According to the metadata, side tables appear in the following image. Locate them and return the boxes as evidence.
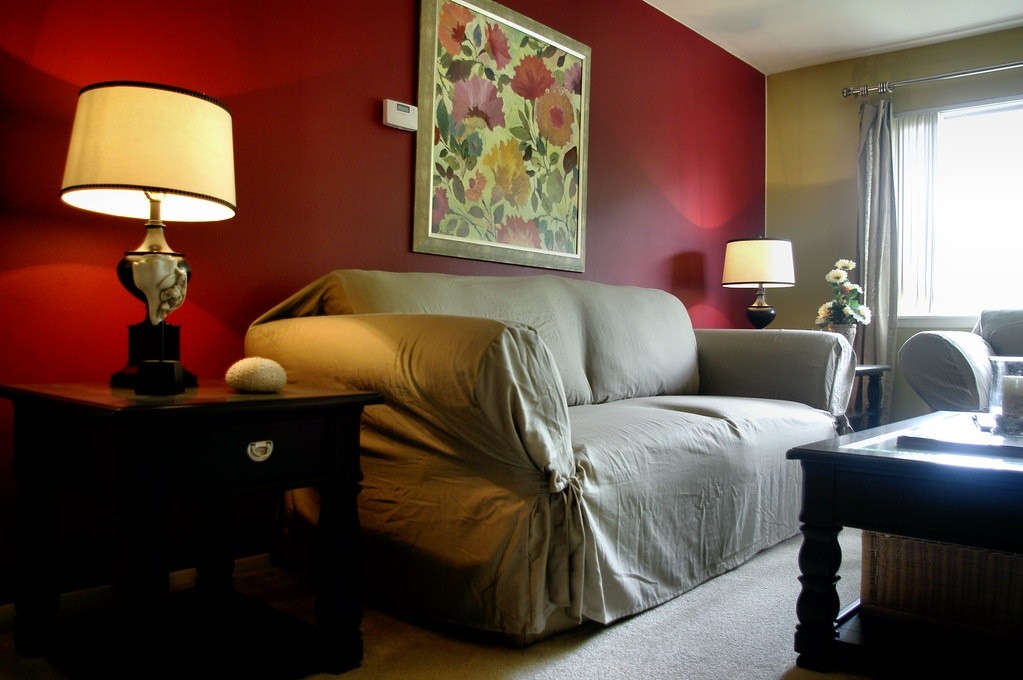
[845,365,892,427]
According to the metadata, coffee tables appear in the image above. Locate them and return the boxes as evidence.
[786,410,1023,679]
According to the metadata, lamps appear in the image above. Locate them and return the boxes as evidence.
[722,238,795,329]
[60,79,237,395]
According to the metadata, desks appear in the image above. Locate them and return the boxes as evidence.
[0,381,386,679]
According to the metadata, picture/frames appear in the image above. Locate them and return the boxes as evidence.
[412,1,590,275]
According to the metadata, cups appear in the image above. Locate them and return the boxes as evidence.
[988,356,1023,420]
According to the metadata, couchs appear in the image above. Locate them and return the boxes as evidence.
[244,269,857,648]
[897,309,1023,415]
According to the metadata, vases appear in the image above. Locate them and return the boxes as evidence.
[827,322,857,349]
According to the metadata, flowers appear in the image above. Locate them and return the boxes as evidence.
[813,258,870,329]
